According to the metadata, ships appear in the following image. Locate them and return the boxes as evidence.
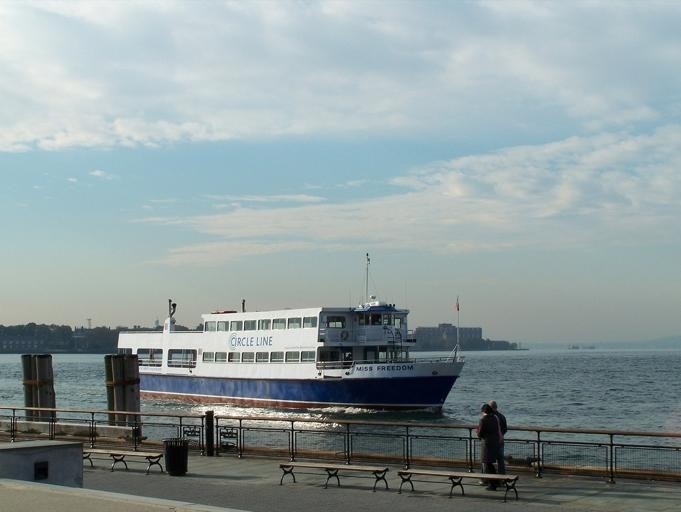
[117,253,468,412]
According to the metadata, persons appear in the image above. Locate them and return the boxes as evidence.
[478,401,507,491]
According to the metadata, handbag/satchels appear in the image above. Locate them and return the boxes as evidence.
[499,431,505,445]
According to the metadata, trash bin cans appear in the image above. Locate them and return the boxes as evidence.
[162,438,189,475]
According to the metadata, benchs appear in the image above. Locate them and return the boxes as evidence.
[279,461,520,502]
[82,449,164,475]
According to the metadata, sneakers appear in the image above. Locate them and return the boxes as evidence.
[479,481,488,486]
[485,486,496,491]
